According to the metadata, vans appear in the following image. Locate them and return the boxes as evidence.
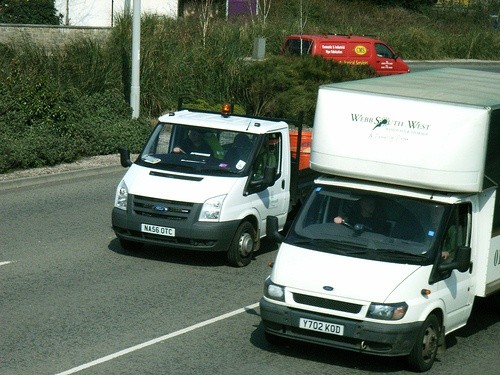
[280,35,410,75]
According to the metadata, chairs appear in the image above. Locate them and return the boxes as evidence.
[202,131,224,161]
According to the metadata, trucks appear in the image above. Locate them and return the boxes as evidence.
[259,65,500,375]
[111,93,323,267]
